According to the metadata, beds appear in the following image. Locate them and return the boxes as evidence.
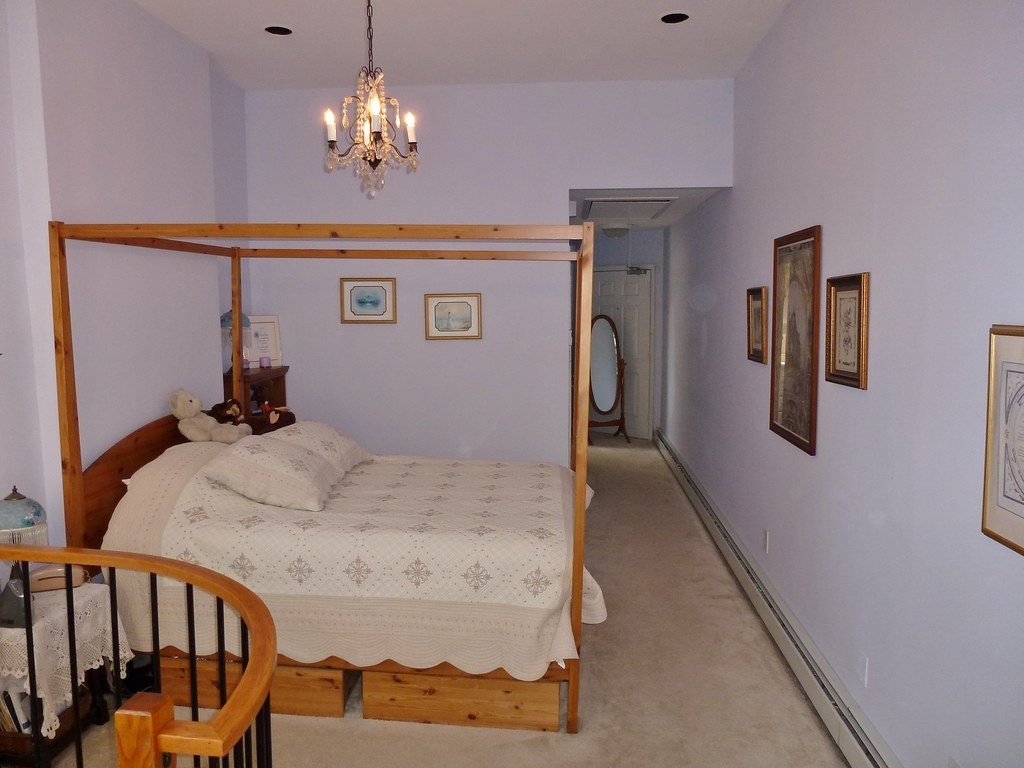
[84,409,609,732]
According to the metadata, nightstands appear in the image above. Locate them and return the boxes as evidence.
[0,582,136,768]
[224,366,290,420]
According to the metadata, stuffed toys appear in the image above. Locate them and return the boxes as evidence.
[201,398,296,436]
[168,389,252,445]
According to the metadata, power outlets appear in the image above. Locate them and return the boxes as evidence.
[763,531,768,553]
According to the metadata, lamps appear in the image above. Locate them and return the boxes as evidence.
[0,484,50,629]
[601,223,630,240]
[321,0,422,200]
[219,309,250,374]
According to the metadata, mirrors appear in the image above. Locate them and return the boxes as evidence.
[588,314,632,447]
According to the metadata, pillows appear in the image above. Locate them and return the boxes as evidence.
[204,418,373,513]
[122,440,229,508]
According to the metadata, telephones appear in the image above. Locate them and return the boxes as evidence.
[28,564,90,593]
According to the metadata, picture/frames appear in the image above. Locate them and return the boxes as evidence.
[424,293,482,340]
[340,277,397,324]
[825,271,870,390]
[745,286,768,365]
[981,324,1024,557]
[769,224,822,457]
